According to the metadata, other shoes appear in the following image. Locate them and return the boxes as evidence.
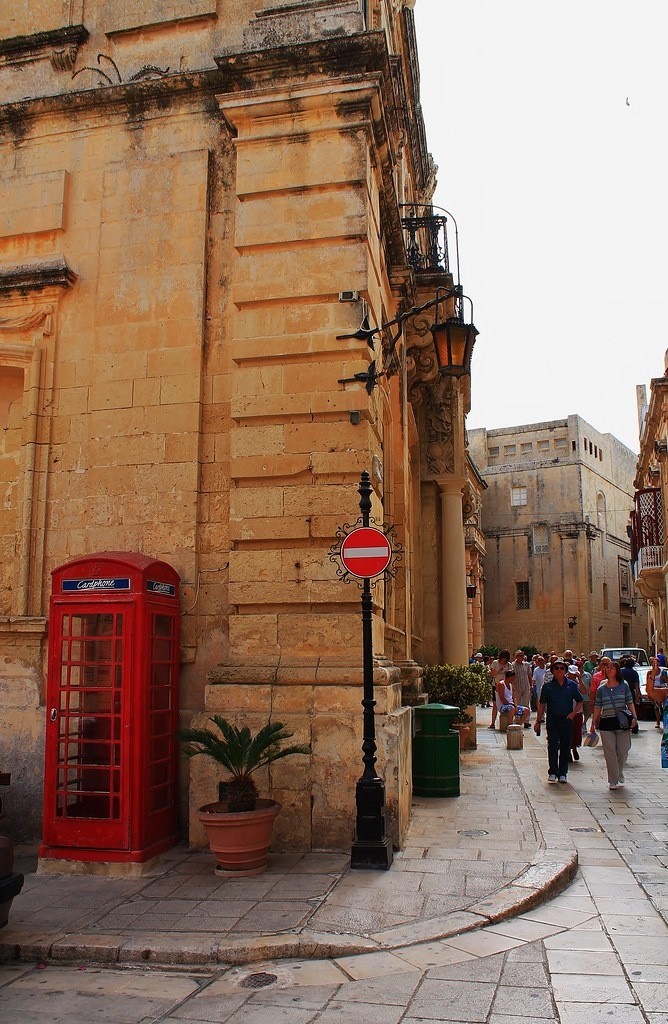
[568,749,579,763]
[548,774,556,783]
[559,775,567,783]
[618,774,624,783]
[524,723,531,727]
[609,782,617,790]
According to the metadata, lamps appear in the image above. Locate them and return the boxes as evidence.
[568,616,577,628]
[466,569,477,599]
[335,282,481,380]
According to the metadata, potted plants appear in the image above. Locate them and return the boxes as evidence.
[175,713,313,876]
[423,660,492,753]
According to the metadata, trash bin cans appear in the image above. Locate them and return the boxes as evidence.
[412,703,460,798]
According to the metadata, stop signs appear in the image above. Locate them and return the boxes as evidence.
[340,526,393,581]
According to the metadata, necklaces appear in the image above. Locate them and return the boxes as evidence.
[611,689,613,691]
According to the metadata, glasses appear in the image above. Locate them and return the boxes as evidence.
[518,655,525,657]
[554,666,565,670]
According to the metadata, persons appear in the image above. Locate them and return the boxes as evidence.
[590,661,637,790]
[534,660,583,782]
[468,647,668,768]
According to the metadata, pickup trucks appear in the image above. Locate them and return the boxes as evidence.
[596,647,668,700]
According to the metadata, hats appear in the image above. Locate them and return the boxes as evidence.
[550,660,568,675]
[568,664,578,674]
[588,651,599,658]
[514,650,524,657]
[474,653,482,658]
[583,732,599,747]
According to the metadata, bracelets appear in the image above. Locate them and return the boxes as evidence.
[537,720,541,723]
[633,717,637,720]
[650,677,655,682]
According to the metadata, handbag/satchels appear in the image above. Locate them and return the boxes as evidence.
[616,710,630,729]
